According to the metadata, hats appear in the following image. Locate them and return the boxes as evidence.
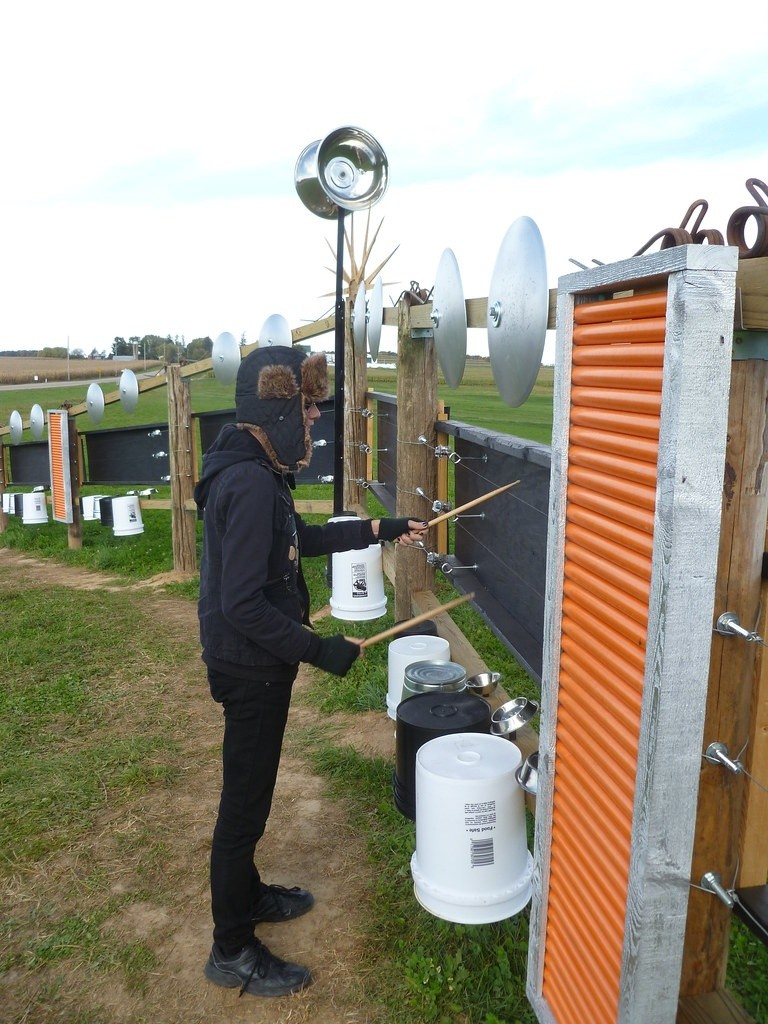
[235,345,328,473]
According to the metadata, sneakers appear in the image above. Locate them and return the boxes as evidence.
[252,881,313,922]
[202,937,311,998]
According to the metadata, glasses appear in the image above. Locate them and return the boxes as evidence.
[304,398,312,412]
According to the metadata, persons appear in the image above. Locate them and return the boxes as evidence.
[194,346,430,997]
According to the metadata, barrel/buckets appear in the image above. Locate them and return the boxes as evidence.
[332,511,358,517]
[1,492,23,517]
[79,494,119,526]
[327,516,362,523]
[392,695,492,822]
[400,660,468,702]
[386,634,450,722]
[393,618,438,640]
[326,553,332,588]
[22,493,49,525]
[328,544,388,621]
[111,495,145,537]
[409,730,535,925]
